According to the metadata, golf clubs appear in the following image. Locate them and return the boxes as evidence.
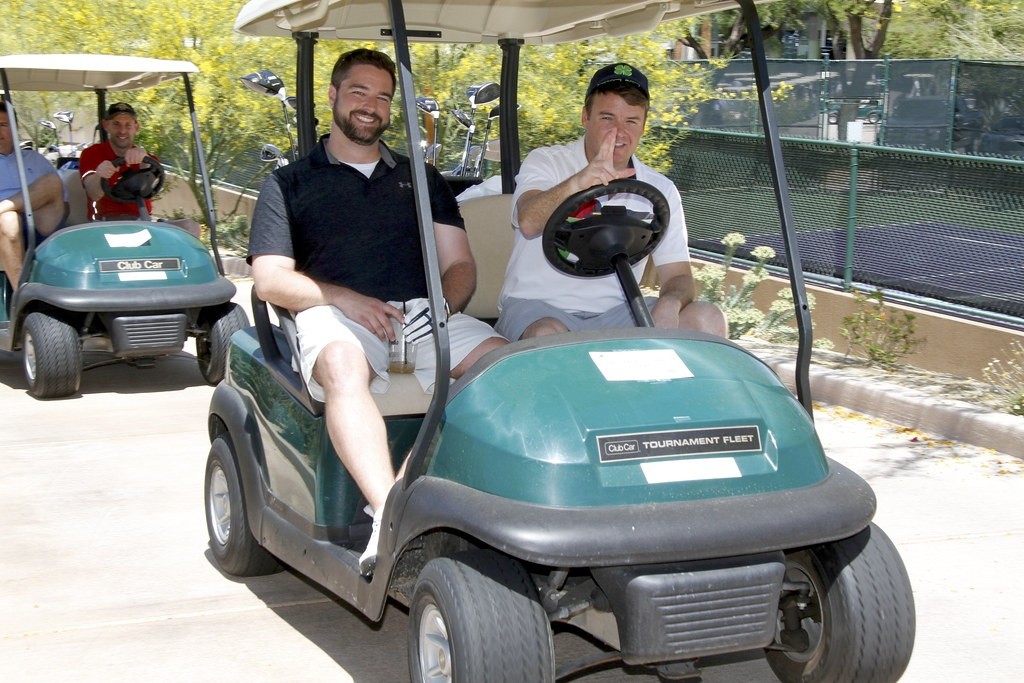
[414,81,522,177]
[39,110,74,158]
[241,70,320,167]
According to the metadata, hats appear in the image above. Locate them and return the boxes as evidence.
[586,63,648,97]
[108,102,135,119]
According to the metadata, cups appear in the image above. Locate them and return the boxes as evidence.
[386,315,416,374]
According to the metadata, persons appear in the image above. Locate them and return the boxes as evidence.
[79,102,201,239]
[0,101,71,292]
[246,49,512,575]
[493,62,729,343]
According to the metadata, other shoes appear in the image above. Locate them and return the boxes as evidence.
[359,502,384,575]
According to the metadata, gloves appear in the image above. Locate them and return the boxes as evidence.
[402,296,451,345]
